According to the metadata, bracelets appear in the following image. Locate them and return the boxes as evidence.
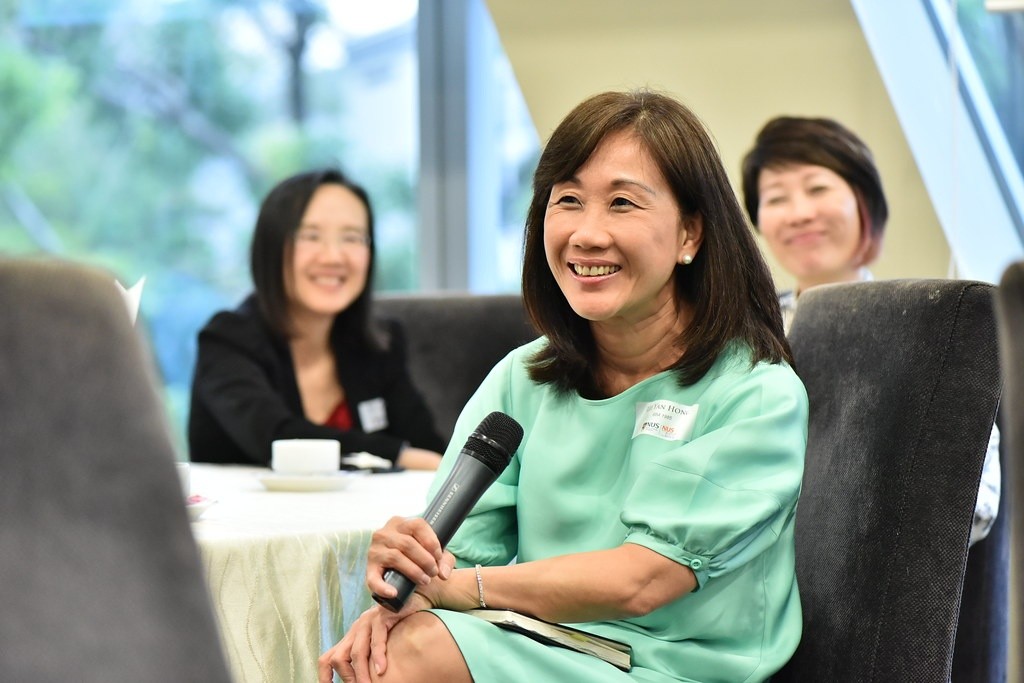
[475,564,488,609]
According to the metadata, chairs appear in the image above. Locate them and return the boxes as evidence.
[0,256,231,683]
[369,293,545,447]
[784,262,1023,683]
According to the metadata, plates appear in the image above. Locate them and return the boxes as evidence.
[179,499,218,522]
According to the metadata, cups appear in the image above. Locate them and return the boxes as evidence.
[271,439,340,477]
[260,476,343,492]
[175,461,191,500]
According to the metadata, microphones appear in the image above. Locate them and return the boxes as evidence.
[370,411,524,613]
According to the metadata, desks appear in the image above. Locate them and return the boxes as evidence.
[175,463,439,683]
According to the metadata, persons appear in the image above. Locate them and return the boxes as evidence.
[187,169,450,475]
[740,116,1003,551]
[317,90,810,683]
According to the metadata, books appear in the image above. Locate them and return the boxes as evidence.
[463,606,633,673]
